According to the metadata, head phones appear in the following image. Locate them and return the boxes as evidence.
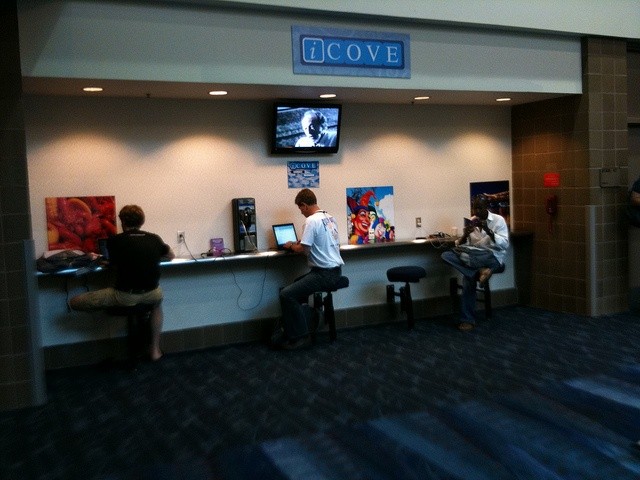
[433,232,445,238]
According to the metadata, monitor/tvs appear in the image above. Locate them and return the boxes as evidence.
[265,98,343,155]
[272,224,298,246]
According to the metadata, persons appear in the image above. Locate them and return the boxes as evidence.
[632,177,640,206]
[348,203,372,245]
[295,110,338,148]
[278,187,345,354]
[442,193,510,333]
[69,204,176,362]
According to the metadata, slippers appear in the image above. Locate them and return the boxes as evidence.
[150,345,166,362]
[479,268,495,288]
[459,322,476,330]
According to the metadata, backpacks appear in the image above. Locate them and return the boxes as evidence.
[36,249,101,275]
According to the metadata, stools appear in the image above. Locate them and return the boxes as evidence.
[476,262,505,321]
[387,266,425,330]
[321,276,349,343]
[121,304,153,364]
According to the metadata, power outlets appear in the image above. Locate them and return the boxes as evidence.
[177,230,185,243]
[416,217,422,228]
